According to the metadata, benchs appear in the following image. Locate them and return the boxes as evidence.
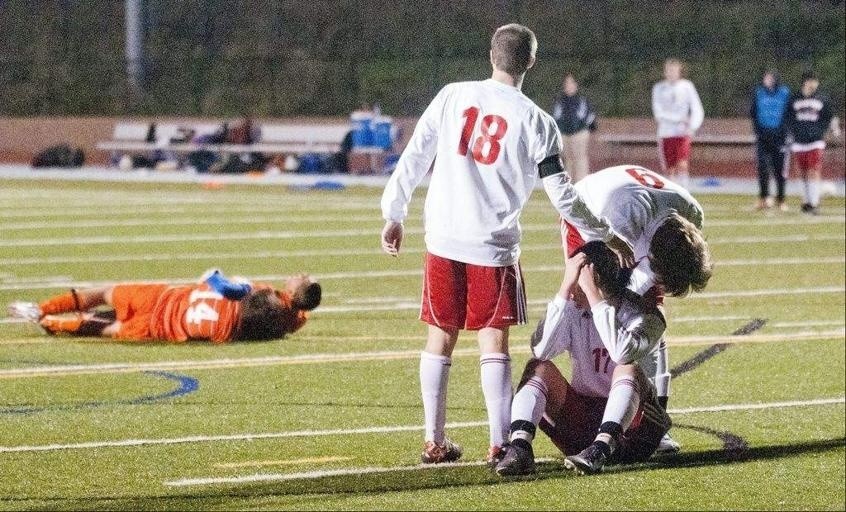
[97,124,352,173]
[593,133,846,185]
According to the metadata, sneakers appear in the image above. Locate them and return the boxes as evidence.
[564,444,606,475]
[486,441,511,467]
[7,301,45,325]
[421,437,464,463]
[495,444,536,475]
[656,432,680,452]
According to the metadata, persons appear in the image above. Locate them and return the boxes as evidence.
[560,161,712,453]
[378,22,637,465]
[490,240,672,478]
[552,68,597,178]
[778,71,841,212]
[652,58,707,177]
[2,281,290,344]
[196,264,323,336]
[364,102,392,151]
[348,99,372,148]
[748,70,794,215]
